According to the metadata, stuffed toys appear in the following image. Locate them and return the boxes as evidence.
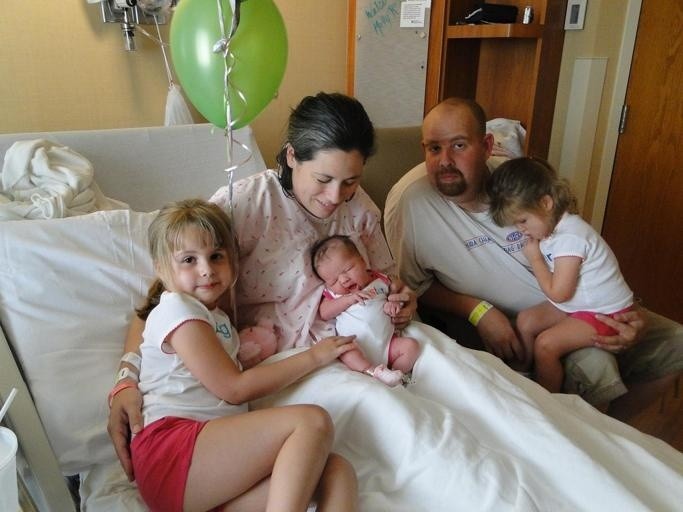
[238,316,279,372]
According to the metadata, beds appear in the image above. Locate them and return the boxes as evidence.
[1,122,683,512]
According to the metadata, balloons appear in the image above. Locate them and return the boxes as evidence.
[168,0,288,135]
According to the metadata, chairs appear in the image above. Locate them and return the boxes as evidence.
[360,126,426,259]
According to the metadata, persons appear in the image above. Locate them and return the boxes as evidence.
[130,196,358,512]
[104,89,417,481]
[310,231,421,387]
[398,96,682,423]
[488,154,635,393]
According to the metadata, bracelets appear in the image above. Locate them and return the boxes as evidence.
[107,381,139,408]
[118,352,142,369]
[113,368,139,383]
[468,301,494,326]
